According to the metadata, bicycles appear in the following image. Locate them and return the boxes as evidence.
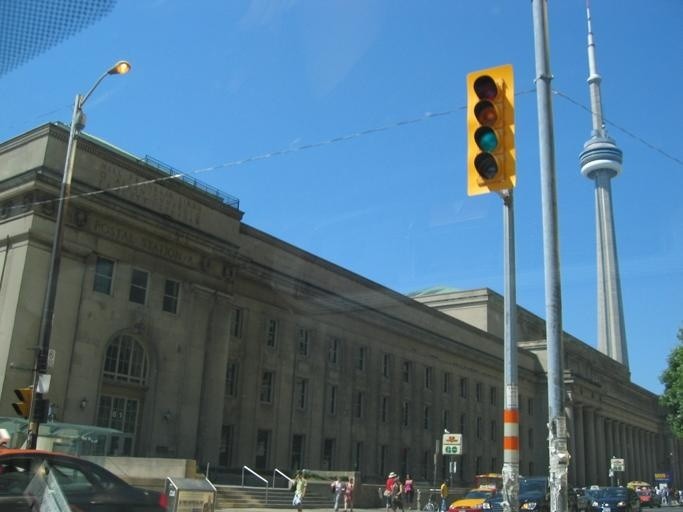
[422,492,438,512]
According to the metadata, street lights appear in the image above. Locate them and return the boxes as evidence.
[24,59,131,452]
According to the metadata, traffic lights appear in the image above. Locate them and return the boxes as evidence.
[11,387,32,419]
[473,74,501,181]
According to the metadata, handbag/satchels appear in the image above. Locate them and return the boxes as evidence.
[384,490,391,496]
[332,486,335,492]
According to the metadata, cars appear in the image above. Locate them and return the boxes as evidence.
[0,448,167,511]
[446,473,661,512]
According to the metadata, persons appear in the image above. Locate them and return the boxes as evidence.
[330,474,346,511]
[655,485,681,506]
[384,472,397,509]
[342,476,355,512]
[439,476,450,512]
[390,476,404,511]
[0,427,11,474]
[291,471,308,512]
[403,472,416,511]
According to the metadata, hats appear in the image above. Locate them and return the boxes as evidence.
[295,470,301,475]
[389,472,397,478]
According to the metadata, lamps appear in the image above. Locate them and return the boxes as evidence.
[79,396,88,409]
